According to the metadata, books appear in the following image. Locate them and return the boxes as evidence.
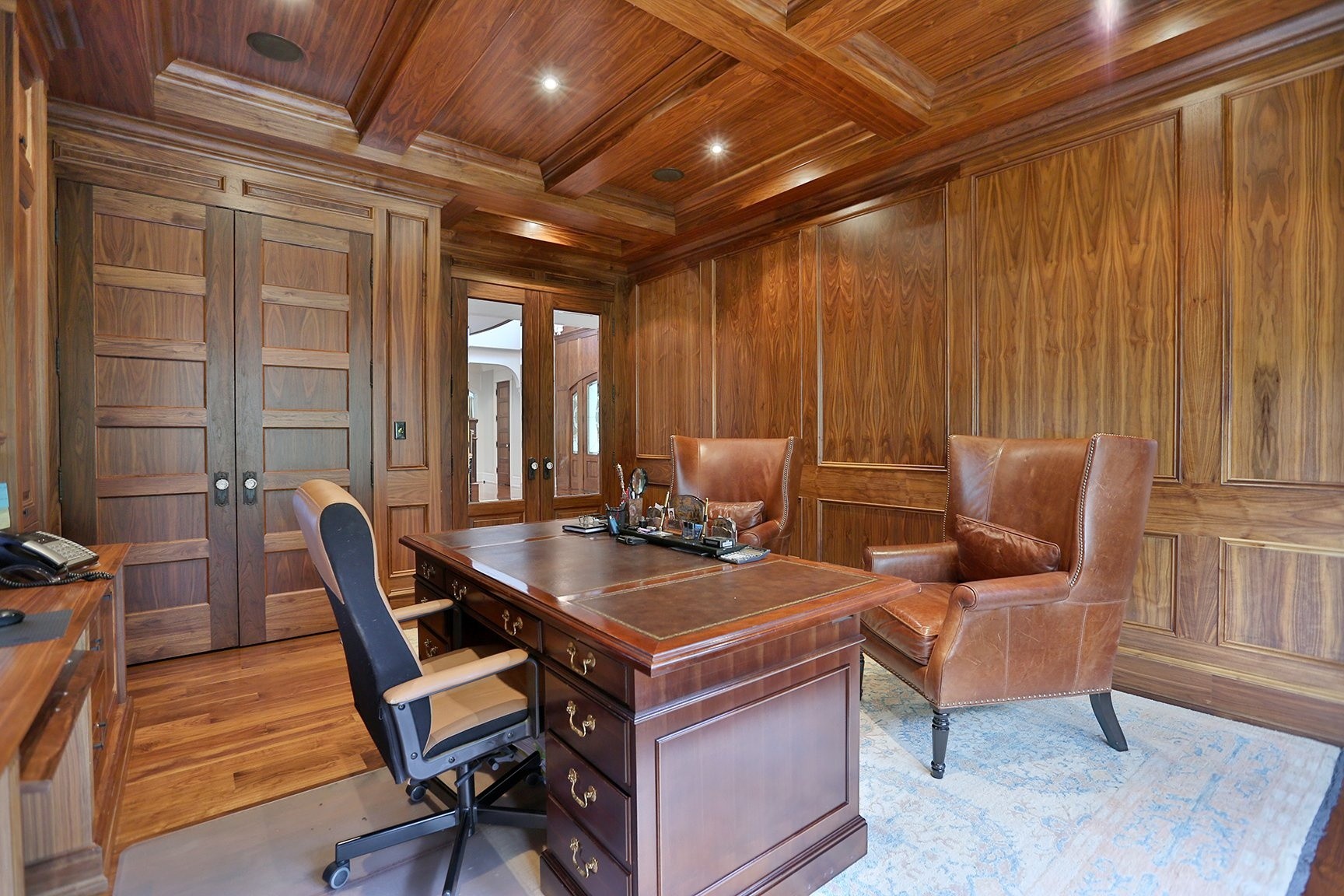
[562,522,606,534]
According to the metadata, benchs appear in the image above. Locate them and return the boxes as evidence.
[392,515,924,896]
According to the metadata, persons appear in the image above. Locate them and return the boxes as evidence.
[669,511,674,520]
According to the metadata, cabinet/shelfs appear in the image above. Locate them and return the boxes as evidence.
[0,539,142,896]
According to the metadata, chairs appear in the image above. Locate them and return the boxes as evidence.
[667,435,809,554]
[290,476,545,896]
[844,431,1164,783]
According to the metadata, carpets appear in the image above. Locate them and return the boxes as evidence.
[122,656,1344,896]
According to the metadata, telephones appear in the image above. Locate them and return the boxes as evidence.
[0,530,100,582]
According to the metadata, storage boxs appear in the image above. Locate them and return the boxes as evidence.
[620,497,643,526]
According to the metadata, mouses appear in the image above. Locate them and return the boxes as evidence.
[0,609,25,627]
